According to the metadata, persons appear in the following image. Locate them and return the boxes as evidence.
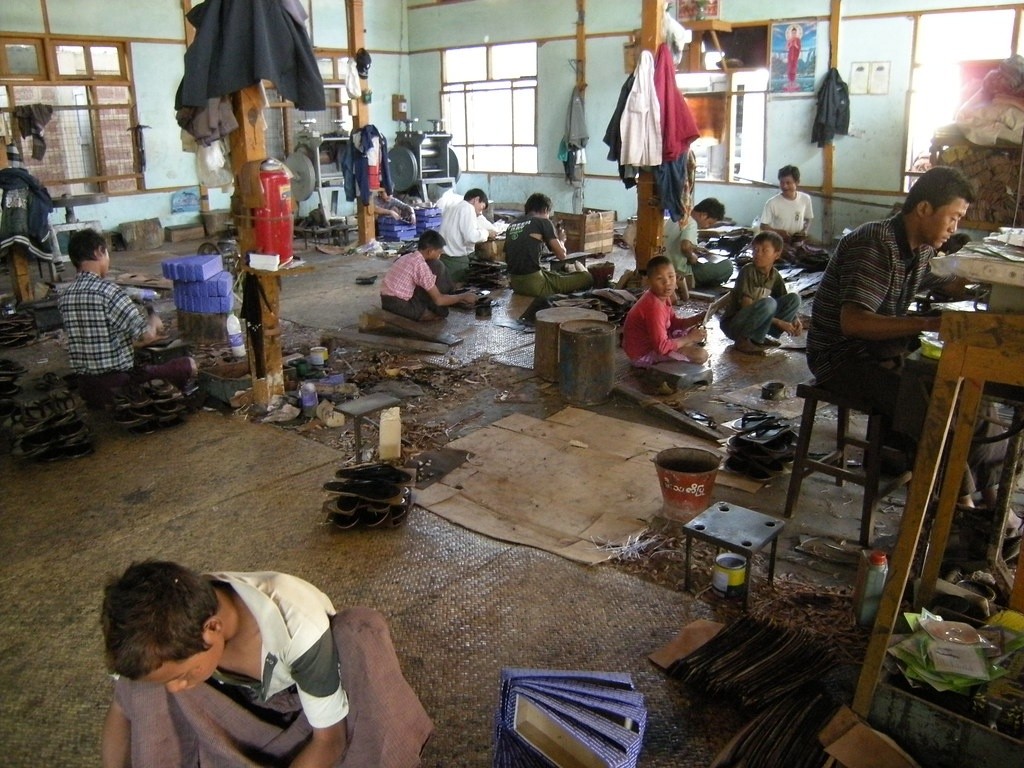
[720,232,803,355]
[623,259,709,368]
[373,180,416,234]
[503,194,592,297]
[663,198,733,289]
[760,165,813,261]
[102,561,432,768]
[380,230,479,321]
[59,227,198,403]
[807,168,1024,561]
[439,189,497,282]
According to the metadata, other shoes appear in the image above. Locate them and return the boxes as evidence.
[1,313,184,466]
[759,337,777,347]
[725,411,798,483]
[736,339,766,354]
[419,308,443,321]
[655,380,672,396]
[1001,517,1023,562]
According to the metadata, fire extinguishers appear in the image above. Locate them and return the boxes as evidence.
[367,136,381,188]
[253,159,293,263]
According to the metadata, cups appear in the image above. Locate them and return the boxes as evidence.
[301,383,318,416]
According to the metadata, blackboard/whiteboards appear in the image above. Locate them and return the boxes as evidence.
[698,20,770,73]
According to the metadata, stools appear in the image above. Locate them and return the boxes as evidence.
[682,500,785,613]
[305,225,356,251]
[783,378,917,549]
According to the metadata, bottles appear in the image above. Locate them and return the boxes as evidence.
[853,549,888,624]
[226,312,247,358]
[126,287,160,301]
[712,552,747,600]
[751,219,760,237]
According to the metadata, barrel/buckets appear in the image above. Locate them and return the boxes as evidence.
[650,448,723,522]
[288,360,309,379]
[557,319,617,408]
[588,264,614,289]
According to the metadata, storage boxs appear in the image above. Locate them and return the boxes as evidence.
[556,208,613,254]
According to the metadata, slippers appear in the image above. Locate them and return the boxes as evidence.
[324,463,406,530]
[686,408,718,428]
